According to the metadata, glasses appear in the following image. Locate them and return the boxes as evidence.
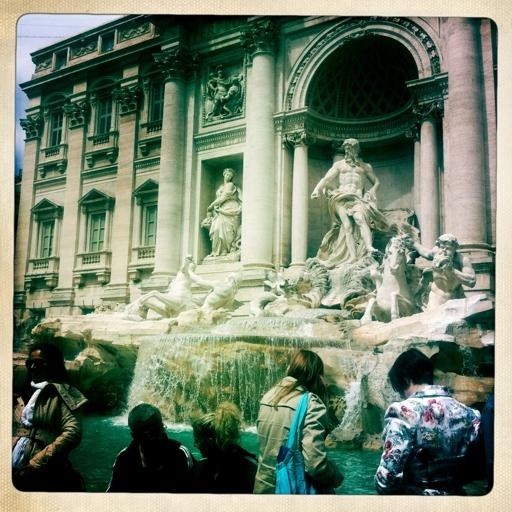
[25,358,47,369]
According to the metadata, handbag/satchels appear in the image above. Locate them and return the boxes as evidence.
[274,447,338,494]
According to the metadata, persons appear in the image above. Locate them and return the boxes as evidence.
[13,343,83,491]
[205,167,241,257]
[400,230,477,311]
[478,360,493,488]
[206,67,241,122]
[187,262,243,327]
[373,348,479,495]
[108,404,188,486]
[183,401,258,492]
[311,136,380,264]
[253,347,343,494]
